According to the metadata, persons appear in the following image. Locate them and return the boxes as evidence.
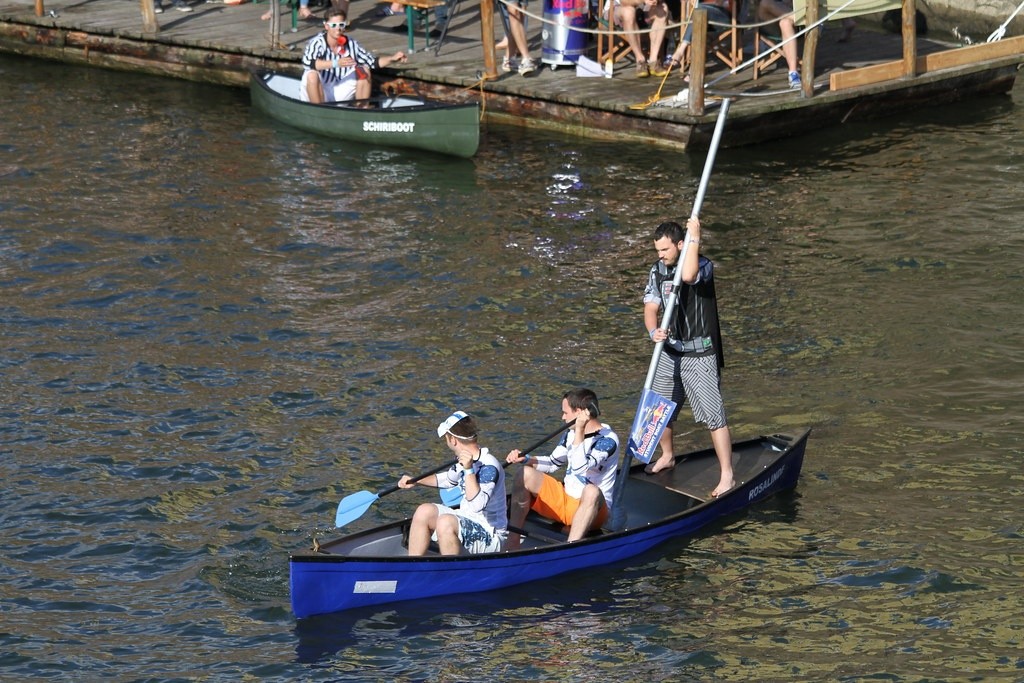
[154,0,193,13]
[494,0,856,89]
[397,411,507,556]
[261,0,449,37]
[505,388,620,550]
[300,7,409,108]
[644,216,736,497]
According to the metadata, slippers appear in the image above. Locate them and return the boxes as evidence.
[375,6,405,17]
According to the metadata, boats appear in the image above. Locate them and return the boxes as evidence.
[248,67,484,161]
[291,421,811,620]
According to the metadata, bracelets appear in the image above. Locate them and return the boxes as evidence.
[520,454,531,464]
[689,239,701,245]
[649,328,658,339]
[464,468,475,476]
[332,59,337,68]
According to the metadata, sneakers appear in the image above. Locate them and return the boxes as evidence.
[788,71,801,89]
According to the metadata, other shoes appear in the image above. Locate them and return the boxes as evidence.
[636,60,649,78]
[392,24,408,32]
[521,58,539,78]
[670,59,681,71]
[502,56,513,72]
[176,1,192,12]
[650,61,670,77]
[154,3,163,13]
[429,28,448,36]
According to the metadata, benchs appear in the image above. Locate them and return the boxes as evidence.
[385,0,446,53]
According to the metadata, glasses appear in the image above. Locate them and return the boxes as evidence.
[323,22,345,29]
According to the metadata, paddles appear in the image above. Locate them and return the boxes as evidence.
[334,459,460,526]
[501,418,576,468]
[607,95,730,535]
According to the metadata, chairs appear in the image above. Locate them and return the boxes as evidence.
[596,0,804,79]
